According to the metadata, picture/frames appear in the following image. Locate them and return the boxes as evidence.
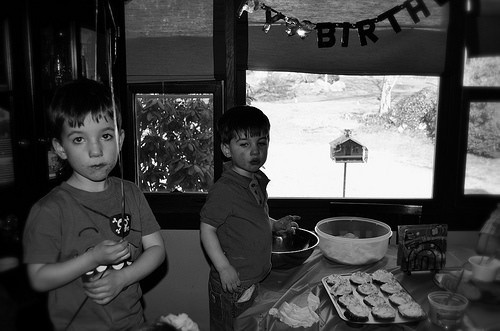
[124,79,224,208]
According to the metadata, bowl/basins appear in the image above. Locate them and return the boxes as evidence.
[314,217,393,266]
[469,256,500,282]
[271,227,319,270]
[428,291,468,328]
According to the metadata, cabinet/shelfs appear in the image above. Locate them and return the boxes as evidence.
[0,0,132,254]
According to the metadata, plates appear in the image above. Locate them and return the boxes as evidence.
[443,278,481,299]
[433,273,460,290]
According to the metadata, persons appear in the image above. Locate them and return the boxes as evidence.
[476,205,500,257]
[200,106,301,331]
[20,79,165,331]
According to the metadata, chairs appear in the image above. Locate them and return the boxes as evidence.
[330,201,422,227]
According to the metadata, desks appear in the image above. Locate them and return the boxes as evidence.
[236,228,500,331]
